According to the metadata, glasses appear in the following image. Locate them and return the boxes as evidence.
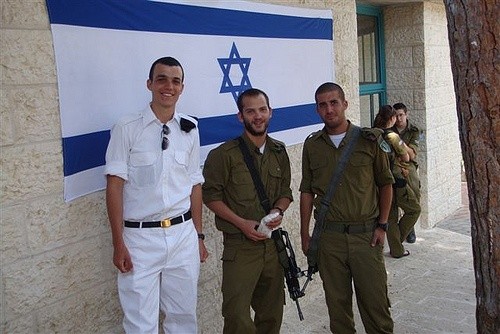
[389,105,395,113]
[160,125,173,150]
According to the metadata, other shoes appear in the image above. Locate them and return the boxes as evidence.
[403,248,410,256]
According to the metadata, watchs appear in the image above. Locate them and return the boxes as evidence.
[376,222,389,231]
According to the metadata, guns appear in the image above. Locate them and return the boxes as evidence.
[254,221,309,322]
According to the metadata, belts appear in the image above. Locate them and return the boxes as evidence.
[124,211,193,228]
[323,222,379,234]
[224,233,246,240]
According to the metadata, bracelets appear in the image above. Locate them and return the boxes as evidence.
[273,207,284,217]
[198,233,205,240]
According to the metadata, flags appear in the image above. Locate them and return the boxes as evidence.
[46,0,334,203]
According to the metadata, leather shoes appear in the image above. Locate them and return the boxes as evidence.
[407,232,416,243]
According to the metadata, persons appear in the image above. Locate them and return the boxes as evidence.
[392,102,423,243]
[104,56,208,333]
[298,81,396,334]
[202,88,294,334]
[372,104,422,259]
